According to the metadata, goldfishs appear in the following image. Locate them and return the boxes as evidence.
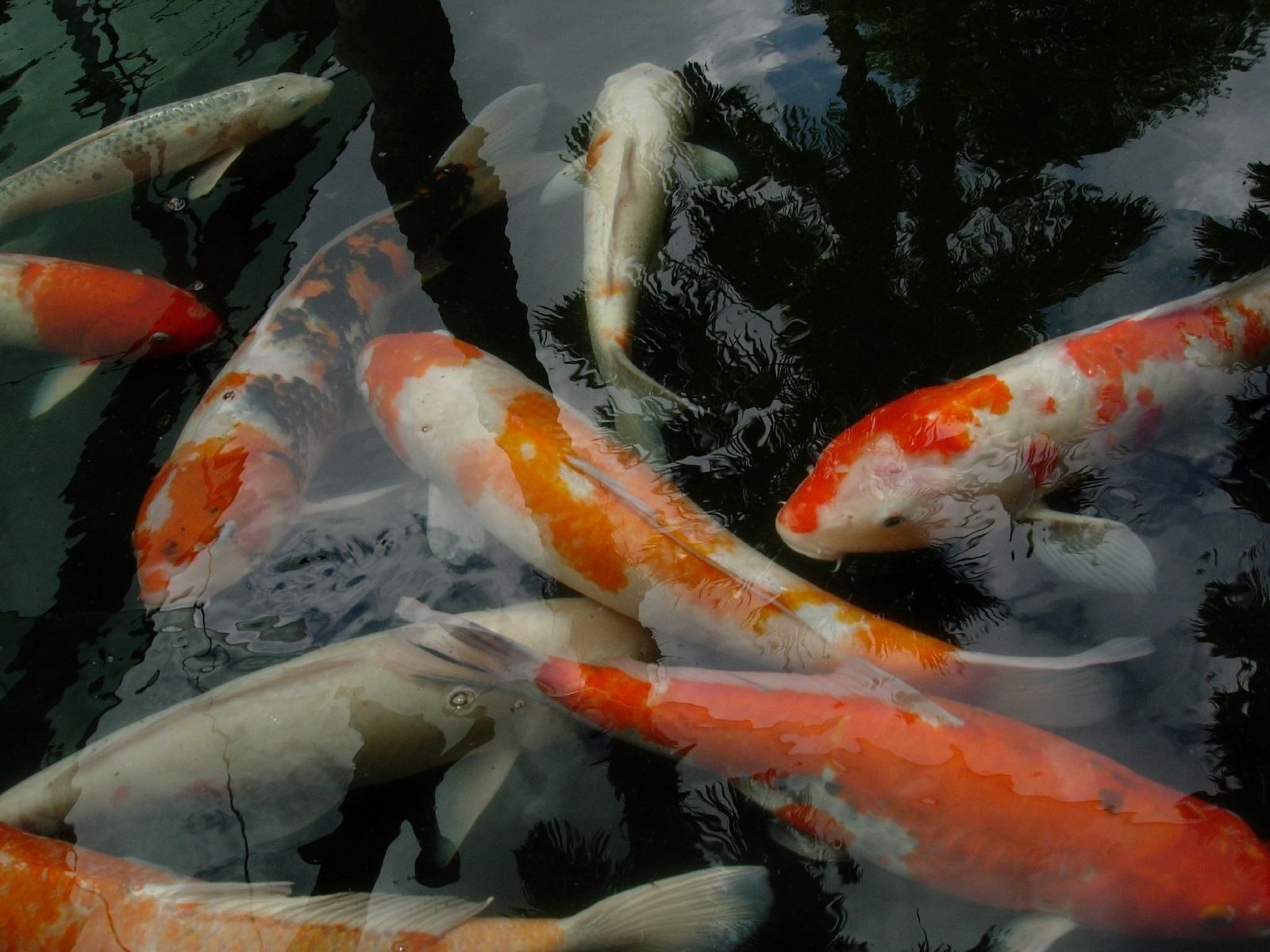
[0,63,1270,952]
